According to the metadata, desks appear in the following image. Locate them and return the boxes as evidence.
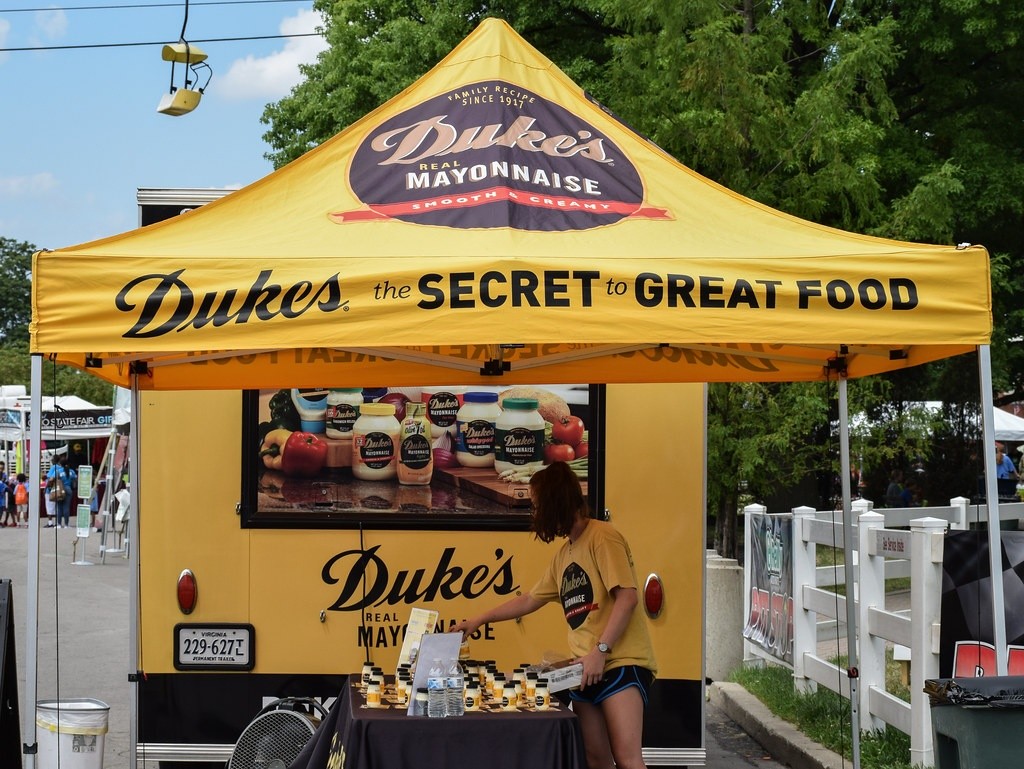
[280,673,587,769]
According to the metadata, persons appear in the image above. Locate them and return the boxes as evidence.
[447,461,658,768]
[995,446,1020,480]
[0,461,6,481]
[46,459,77,528]
[850,463,859,500]
[900,478,918,507]
[13,473,28,527]
[0,472,13,523]
[86,480,98,527]
[1019,448,1024,482]
[1,480,16,527]
[40,475,47,487]
[43,454,62,527]
[886,469,904,496]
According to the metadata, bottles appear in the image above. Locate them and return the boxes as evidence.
[457,631,471,660]
[287,386,546,487]
[358,656,551,717]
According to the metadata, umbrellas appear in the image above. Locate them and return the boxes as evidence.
[828,401,1024,442]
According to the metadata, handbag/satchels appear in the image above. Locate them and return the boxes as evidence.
[48,479,66,503]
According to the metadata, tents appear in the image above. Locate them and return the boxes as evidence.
[23,19,1009,769]
[0,395,113,527]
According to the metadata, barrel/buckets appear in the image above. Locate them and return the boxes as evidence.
[35,695,110,769]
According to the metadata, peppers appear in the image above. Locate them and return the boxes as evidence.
[259,390,328,482]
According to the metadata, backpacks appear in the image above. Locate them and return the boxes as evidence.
[15,484,27,504]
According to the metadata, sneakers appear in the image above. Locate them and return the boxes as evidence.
[1,521,16,527]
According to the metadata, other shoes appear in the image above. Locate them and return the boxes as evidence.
[54,524,71,529]
[17,522,29,528]
[44,524,53,527]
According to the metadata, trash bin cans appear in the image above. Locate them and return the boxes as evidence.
[36,696,110,769]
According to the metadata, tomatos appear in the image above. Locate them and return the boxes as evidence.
[544,417,588,463]
